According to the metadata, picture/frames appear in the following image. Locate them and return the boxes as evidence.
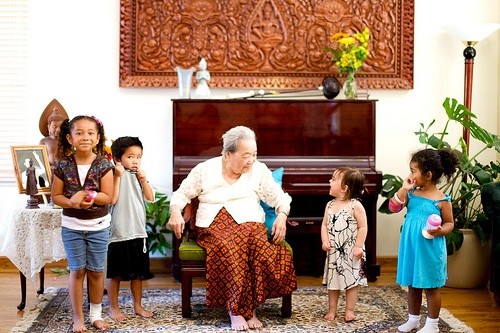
[10,145,53,194]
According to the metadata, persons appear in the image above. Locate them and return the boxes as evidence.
[106,136,155,320]
[320,166,368,322]
[26,159,38,199]
[389,148,461,333]
[39,99,69,183]
[196,58,210,97]
[169,125,297,330]
[51,116,116,332]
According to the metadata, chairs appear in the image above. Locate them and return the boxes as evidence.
[173,170,293,318]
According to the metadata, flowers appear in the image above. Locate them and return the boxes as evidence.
[92,116,103,127]
[326,26,371,96]
[93,145,112,161]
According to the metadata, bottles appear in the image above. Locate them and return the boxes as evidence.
[422,214,442,240]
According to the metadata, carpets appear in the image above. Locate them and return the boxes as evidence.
[9,286,474,333]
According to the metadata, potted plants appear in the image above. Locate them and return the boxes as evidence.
[379,96,500,288]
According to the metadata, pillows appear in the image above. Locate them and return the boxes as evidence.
[260,167,284,239]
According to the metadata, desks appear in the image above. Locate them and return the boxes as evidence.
[0,204,107,309]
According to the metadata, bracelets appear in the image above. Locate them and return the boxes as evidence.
[279,211,288,216]
[394,192,406,204]
[85,190,97,203]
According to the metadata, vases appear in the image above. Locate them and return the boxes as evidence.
[343,70,358,98]
